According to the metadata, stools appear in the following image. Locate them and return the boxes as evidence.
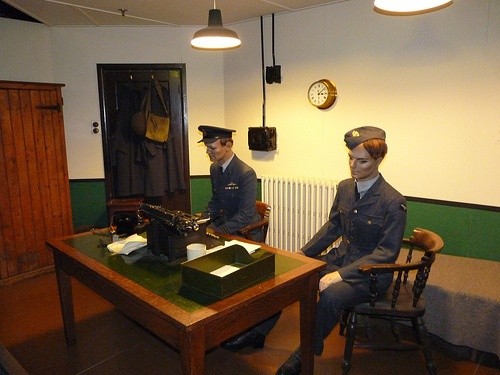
[107,198,144,226]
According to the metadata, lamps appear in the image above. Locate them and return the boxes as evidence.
[373,0,455,16]
[191,0,242,52]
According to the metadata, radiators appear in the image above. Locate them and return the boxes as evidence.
[261,175,342,256]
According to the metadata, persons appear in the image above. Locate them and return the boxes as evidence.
[198,125,264,242]
[220,126,408,375]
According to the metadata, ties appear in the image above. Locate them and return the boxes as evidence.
[355,182,360,202]
[219,167,223,178]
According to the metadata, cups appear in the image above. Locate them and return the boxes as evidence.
[186,244,206,260]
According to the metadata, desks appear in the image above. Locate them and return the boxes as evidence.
[45,217,327,375]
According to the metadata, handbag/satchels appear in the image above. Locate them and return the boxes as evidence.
[130,71,170,142]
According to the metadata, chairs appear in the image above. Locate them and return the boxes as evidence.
[195,200,271,244]
[340,228,444,375]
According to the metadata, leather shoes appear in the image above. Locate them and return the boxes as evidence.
[220,329,264,351]
[275,348,302,375]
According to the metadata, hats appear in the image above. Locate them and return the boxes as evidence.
[197,125,236,143]
[344,126,386,150]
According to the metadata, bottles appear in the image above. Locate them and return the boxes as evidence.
[116,216,134,236]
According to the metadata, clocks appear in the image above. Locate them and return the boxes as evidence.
[308,79,337,110]
